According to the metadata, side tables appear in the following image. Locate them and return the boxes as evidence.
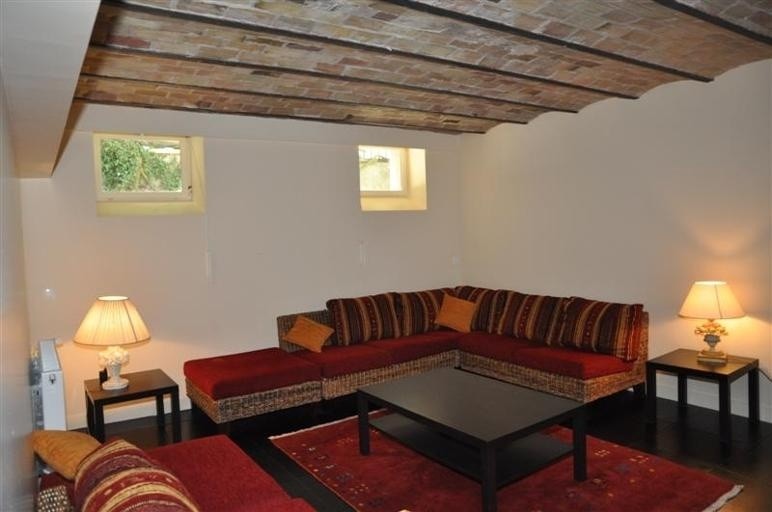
[646,348,760,442]
[84,368,182,445]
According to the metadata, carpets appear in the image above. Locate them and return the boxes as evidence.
[269,409,745,512]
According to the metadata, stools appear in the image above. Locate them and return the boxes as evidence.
[183,347,322,424]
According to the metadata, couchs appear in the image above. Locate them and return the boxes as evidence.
[276,286,649,421]
[36,435,316,512]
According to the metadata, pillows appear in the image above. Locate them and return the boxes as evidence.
[325,291,400,343]
[432,291,476,334]
[463,284,643,362]
[388,284,456,336]
[282,313,335,353]
[28,430,201,512]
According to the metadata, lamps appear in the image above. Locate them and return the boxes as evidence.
[73,295,150,390]
[677,281,745,355]
